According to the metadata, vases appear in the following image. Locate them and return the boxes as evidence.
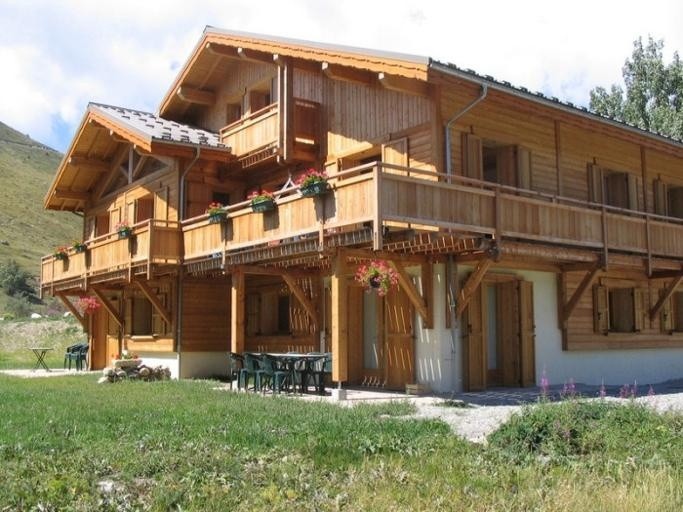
[301,185,328,196]
[370,274,381,287]
[209,215,227,224]
[76,246,84,251]
[251,201,274,214]
[57,254,64,260]
[118,231,131,239]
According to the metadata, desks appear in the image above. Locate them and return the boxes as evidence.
[28,347,54,372]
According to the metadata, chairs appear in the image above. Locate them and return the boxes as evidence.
[231,349,332,395]
[64,343,82,371]
[69,343,89,371]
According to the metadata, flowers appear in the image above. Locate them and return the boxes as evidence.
[72,239,87,248]
[205,202,227,215]
[297,168,330,185]
[54,247,66,256]
[247,190,275,205]
[114,220,132,232]
[354,261,401,299]
